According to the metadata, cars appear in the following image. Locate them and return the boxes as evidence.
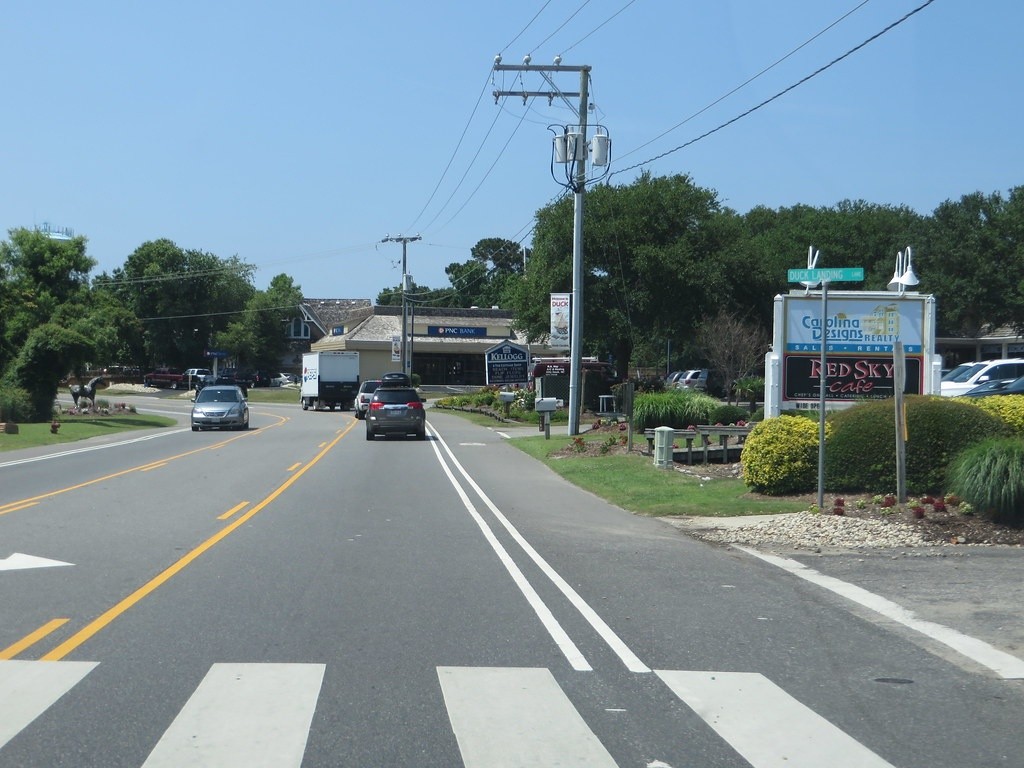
[190,384,251,432]
[938,356,1024,400]
[215,367,302,389]
[666,368,756,401]
[354,379,382,421]
[182,369,212,382]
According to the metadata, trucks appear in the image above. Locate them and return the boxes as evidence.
[297,351,358,412]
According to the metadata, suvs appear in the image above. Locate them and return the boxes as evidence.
[362,383,426,441]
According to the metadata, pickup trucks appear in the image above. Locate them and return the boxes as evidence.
[143,367,198,390]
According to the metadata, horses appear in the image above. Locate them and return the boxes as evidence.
[69,376,106,406]
